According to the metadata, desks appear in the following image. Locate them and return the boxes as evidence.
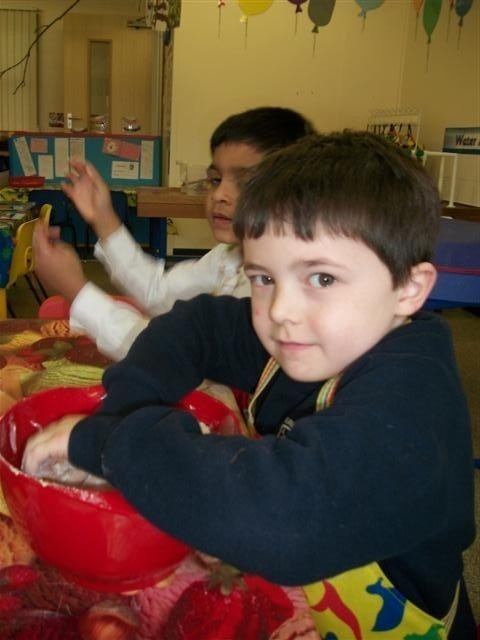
[137,184,214,262]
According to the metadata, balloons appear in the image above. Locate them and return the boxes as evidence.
[236,0,479,47]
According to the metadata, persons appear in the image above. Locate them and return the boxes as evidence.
[19,129,479,639]
[30,106,320,366]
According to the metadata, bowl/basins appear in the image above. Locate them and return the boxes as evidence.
[1,383,244,595]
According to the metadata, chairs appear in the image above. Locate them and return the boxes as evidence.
[6,203,54,320]
